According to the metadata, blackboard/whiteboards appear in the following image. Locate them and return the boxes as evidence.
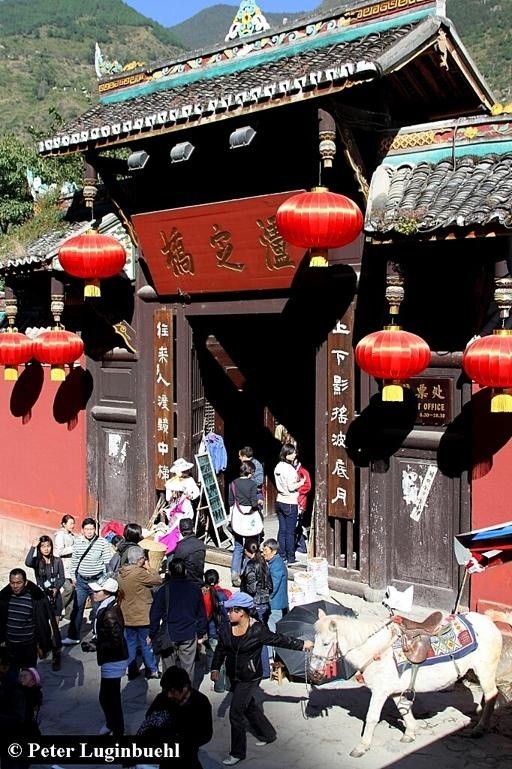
[194,451,228,529]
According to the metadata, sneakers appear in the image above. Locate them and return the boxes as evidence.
[221,748,247,765]
[59,634,82,645]
[255,736,277,747]
[231,570,244,588]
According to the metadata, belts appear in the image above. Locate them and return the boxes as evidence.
[76,571,103,582]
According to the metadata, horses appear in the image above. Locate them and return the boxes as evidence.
[308,608,503,759]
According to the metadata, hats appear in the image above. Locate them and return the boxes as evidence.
[168,458,195,473]
[86,575,120,594]
[218,589,256,610]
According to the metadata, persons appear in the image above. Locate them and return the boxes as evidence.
[160,457,201,527]
[1,511,314,769]
[237,445,266,496]
[227,459,266,588]
[273,443,299,565]
[292,449,312,554]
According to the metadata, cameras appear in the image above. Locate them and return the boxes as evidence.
[44,585,55,603]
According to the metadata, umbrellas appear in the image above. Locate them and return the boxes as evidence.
[273,600,360,686]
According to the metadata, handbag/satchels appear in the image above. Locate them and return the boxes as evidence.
[230,499,264,537]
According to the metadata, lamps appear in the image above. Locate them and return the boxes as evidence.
[125,140,159,176]
[227,123,254,149]
[168,135,196,164]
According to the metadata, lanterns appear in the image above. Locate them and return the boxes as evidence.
[351,322,436,401]
[29,323,89,381]
[56,229,128,298]
[462,326,512,414]
[271,184,365,268]
[0,325,35,382]
